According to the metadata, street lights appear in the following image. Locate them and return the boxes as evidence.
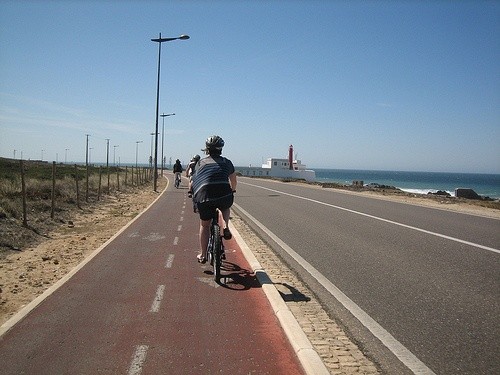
[150,32,190,192]
[150,132,160,169]
[159,112,175,178]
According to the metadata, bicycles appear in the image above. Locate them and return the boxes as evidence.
[197,190,237,283]
[175,173,181,189]
[187,176,197,213]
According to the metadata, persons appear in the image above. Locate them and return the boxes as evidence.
[185,154,200,198]
[190,136,236,264]
[173,158,182,187]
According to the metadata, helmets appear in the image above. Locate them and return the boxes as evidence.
[205,135,225,153]
[193,154,200,160]
[176,159,179,163]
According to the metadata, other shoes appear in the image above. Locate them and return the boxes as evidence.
[197,254,207,263]
[223,227,232,240]
